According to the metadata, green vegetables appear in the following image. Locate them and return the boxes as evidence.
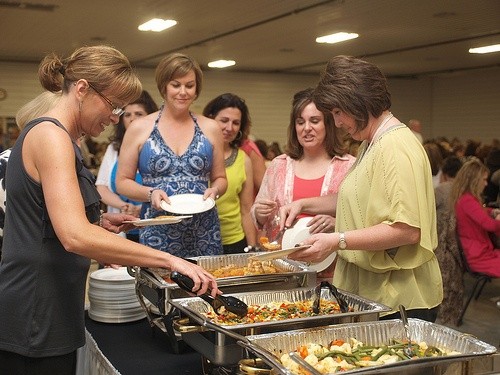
[205,303,313,322]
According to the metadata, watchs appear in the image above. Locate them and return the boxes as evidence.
[338,233,347,250]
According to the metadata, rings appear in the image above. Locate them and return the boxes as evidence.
[322,223,326,228]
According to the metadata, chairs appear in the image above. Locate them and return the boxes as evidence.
[456,229,497,326]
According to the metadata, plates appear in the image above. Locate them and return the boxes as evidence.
[160,193,215,214]
[281,217,336,273]
[87,266,164,324]
[122,214,193,226]
[247,244,311,262]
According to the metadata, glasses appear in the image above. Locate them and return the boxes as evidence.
[74,81,126,117]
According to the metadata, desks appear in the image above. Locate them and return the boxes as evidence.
[76,304,205,375]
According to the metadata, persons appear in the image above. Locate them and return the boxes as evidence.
[115,54,228,259]
[277,54,444,324]
[0,90,84,260]
[408,119,500,326]
[0,45,223,375]
[95,89,159,244]
[202,94,284,254]
[251,88,357,284]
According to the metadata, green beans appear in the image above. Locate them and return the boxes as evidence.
[316,343,442,366]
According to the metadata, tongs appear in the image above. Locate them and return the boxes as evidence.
[312,281,349,315]
[170,271,248,318]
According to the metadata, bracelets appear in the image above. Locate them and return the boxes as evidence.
[148,188,159,201]
[98,210,104,226]
